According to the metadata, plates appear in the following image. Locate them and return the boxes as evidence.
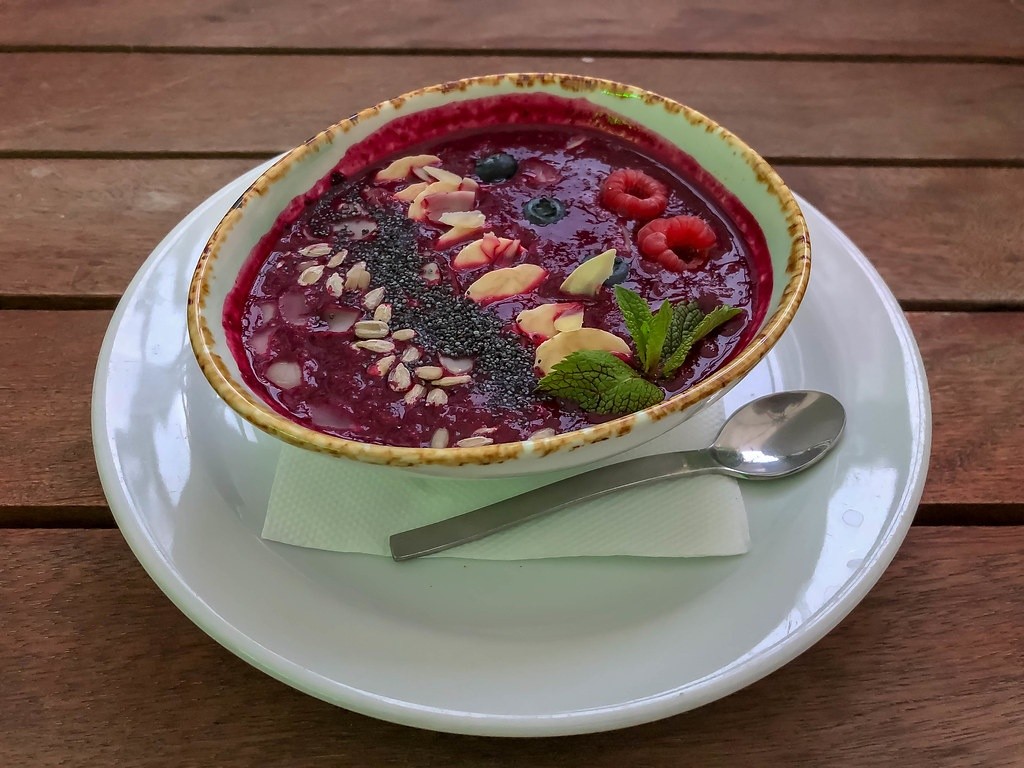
[88,151,931,736]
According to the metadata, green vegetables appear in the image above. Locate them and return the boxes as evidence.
[534,288,743,414]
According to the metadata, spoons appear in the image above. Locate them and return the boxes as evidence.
[388,389,845,559]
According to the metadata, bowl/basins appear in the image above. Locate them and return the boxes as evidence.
[186,70,812,479]
